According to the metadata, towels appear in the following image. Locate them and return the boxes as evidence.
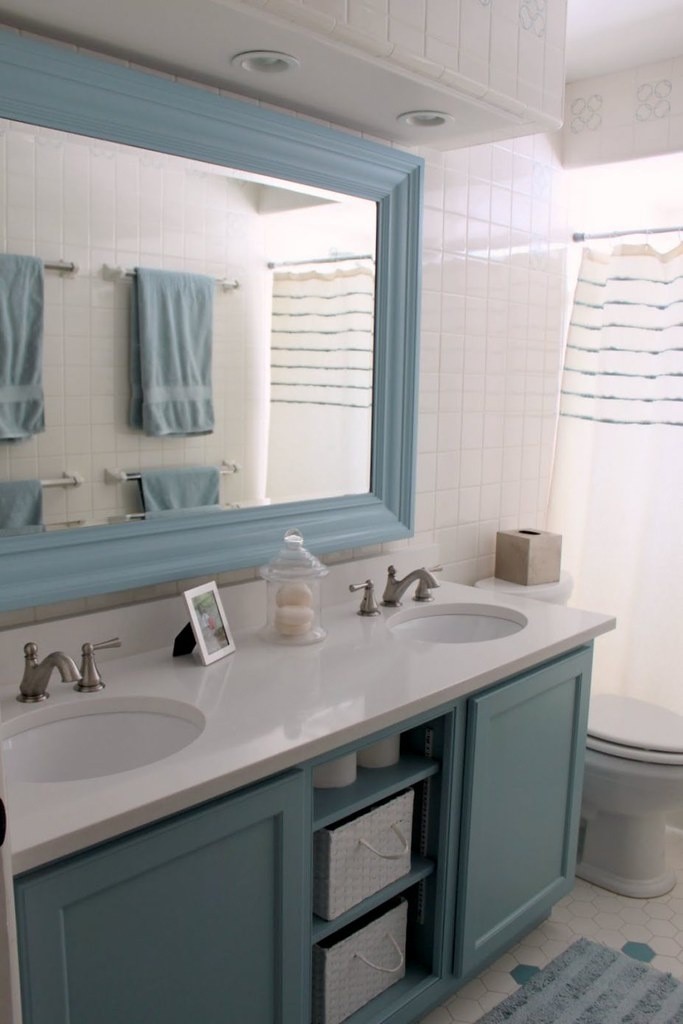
[139,502,226,520]
[131,464,222,514]
[0,251,46,447]
[0,524,48,539]
[130,266,216,437]
[0,479,43,525]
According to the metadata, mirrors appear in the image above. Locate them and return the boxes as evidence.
[0,31,427,613]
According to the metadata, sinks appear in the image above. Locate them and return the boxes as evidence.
[0,694,208,785]
[385,600,530,644]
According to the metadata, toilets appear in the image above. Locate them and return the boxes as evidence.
[471,566,683,900]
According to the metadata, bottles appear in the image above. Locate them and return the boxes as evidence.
[258,527,330,645]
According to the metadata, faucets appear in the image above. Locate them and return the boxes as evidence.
[380,564,442,607]
[15,642,85,704]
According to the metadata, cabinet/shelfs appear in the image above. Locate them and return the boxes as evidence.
[14,640,594,1024]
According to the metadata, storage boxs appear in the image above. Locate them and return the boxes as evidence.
[494,528,562,585]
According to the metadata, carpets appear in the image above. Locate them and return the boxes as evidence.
[474,938,683,1024]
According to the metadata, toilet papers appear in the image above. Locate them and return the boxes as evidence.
[358,733,401,767]
[313,750,358,790]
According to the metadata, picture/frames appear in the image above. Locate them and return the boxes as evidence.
[173,581,236,667]
